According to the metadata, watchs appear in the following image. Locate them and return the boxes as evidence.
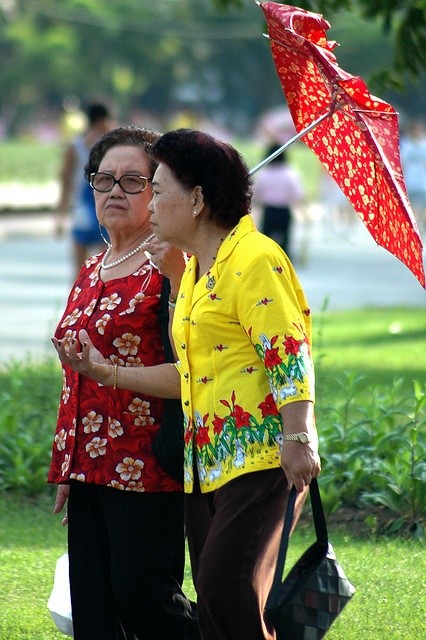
[283,432,316,445]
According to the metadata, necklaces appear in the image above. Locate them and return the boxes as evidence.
[99,232,155,270]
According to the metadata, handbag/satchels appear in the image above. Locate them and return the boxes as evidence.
[262,477,356,640]
[152,275,183,485]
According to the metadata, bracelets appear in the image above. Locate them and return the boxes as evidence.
[111,364,119,391]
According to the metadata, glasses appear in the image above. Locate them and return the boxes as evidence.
[89,172,153,195]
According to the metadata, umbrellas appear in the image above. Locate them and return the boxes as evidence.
[246,0,426,291]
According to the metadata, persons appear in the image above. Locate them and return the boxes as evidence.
[56,106,111,270]
[51,131,322,639]
[49,127,200,639]
[251,138,300,252]
